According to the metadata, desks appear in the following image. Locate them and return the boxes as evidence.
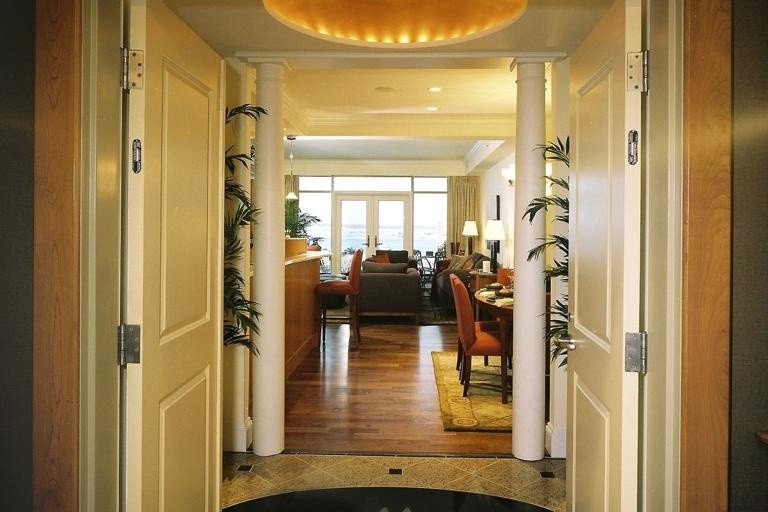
[474,288,512,404]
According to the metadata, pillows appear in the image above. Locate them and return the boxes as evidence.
[362,251,409,273]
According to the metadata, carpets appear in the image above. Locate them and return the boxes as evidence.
[431,351,512,433]
[322,296,457,325]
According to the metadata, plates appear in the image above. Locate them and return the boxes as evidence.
[483,284,503,290]
[494,292,513,297]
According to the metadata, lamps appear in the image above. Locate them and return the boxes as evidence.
[462,221,479,256]
[484,219,506,273]
[286,136,298,200]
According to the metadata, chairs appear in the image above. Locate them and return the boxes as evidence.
[315,250,363,347]
[351,268,420,325]
[449,269,513,397]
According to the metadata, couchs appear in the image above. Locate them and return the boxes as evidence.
[437,253,499,310]
